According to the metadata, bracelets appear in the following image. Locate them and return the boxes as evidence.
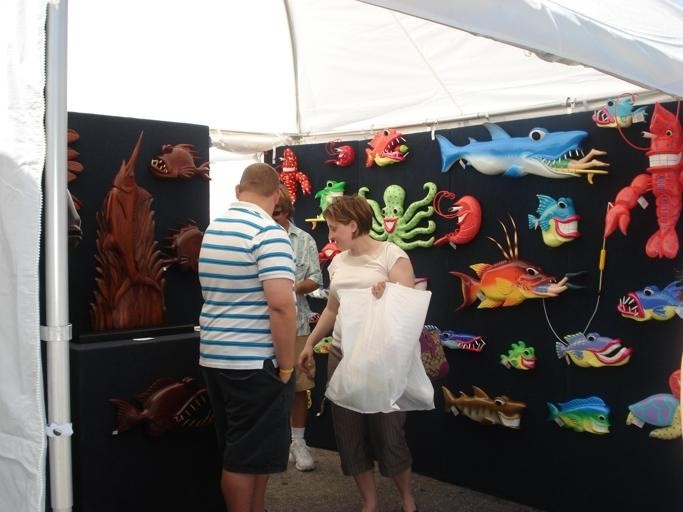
[279,368,294,374]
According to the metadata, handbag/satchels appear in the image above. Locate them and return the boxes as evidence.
[393,342,435,411]
[324,282,432,414]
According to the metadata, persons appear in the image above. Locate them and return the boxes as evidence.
[271,180,324,472]
[198,162,298,511]
[297,195,417,512]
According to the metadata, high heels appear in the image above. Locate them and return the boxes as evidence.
[401,503,418,512]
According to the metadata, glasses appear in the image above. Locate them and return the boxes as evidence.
[273,210,283,216]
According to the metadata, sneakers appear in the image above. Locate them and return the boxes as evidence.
[289,439,315,471]
